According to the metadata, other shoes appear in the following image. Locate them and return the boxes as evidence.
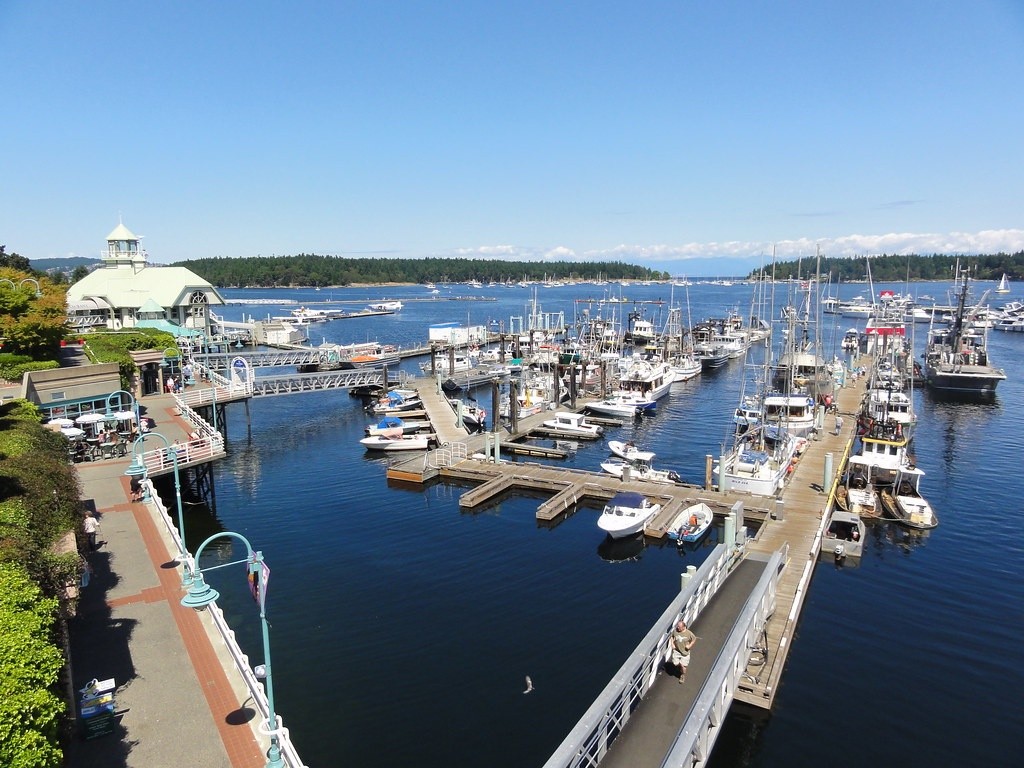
[679,674,685,684]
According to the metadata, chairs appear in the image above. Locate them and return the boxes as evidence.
[74,429,145,462]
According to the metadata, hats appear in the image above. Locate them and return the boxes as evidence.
[86,511,92,515]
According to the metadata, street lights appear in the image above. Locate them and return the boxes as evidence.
[186,362,217,433]
[103,391,147,482]
[181,532,288,768]
[159,347,187,418]
[177,323,229,381]
[124,432,194,585]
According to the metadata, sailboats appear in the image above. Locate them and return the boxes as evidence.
[419,244,1023,528]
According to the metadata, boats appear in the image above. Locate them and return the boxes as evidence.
[349,385,436,450]
[597,492,660,539]
[226,301,404,372]
[821,511,866,557]
[666,503,713,543]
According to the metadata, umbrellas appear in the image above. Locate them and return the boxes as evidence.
[47,418,85,442]
[107,410,136,430]
[75,413,104,436]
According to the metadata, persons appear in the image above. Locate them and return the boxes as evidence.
[95,430,105,449]
[74,440,86,455]
[128,423,138,442]
[862,364,867,373]
[83,511,100,554]
[835,415,844,435]
[670,622,696,674]
[167,376,174,392]
[851,372,857,387]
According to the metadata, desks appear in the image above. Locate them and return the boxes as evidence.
[100,443,116,448]
[119,432,131,438]
[86,438,99,442]
